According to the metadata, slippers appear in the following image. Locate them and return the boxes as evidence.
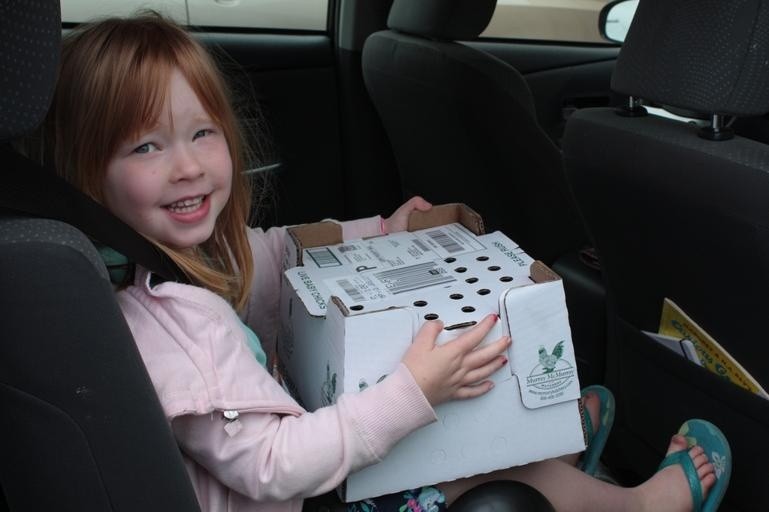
[578,386,614,473]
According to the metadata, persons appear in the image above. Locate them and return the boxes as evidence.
[28,8,733,512]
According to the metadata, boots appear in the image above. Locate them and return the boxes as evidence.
[659,421,729,512]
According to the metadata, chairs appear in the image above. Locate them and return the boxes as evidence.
[360,0,562,247]
[0,2,204,512]
[548,1,768,510]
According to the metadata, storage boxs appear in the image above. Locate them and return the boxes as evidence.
[296,202,592,504]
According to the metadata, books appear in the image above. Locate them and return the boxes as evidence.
[657,297,767,400]
[640,330,704,366]
